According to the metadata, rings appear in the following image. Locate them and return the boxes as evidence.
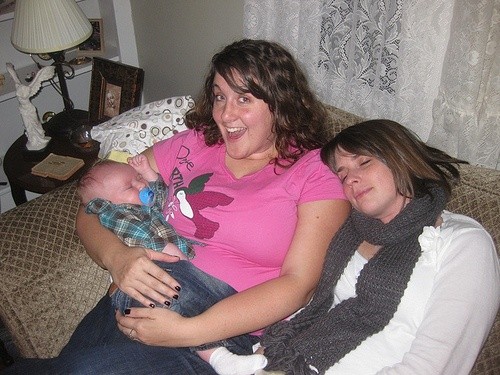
[128,329,134,341]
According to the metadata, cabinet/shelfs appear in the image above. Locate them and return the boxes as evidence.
[0,0,145,206]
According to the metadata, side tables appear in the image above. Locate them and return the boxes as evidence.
[3,111,101,206]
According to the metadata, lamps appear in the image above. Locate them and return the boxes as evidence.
[10,0,93,135]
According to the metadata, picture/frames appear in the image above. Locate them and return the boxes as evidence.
[78,18,104,55]
[89,56,145,128]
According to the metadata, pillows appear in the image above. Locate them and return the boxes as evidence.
[91,95,195,159]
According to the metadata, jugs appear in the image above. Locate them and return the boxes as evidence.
[64,118,101,155]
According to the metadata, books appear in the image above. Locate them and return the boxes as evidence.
[30,152,83,180]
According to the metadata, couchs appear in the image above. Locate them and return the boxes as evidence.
[0,103,500,375]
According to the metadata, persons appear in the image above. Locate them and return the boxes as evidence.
[0,38,352,375]
[76,156,268,374]
[250,120,500,374]
